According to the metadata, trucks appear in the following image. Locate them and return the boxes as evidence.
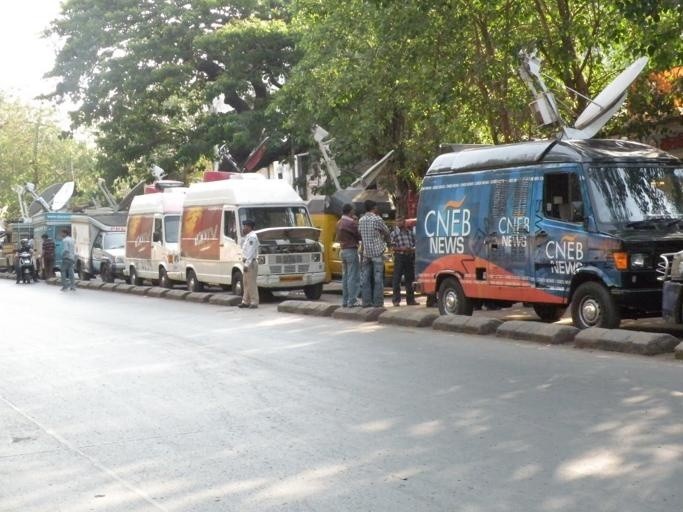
[411,48,682,330]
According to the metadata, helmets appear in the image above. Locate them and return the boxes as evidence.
[21,238,30,248]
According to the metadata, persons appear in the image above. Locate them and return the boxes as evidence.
[58,229,77,292]
[12,234,56,282]
[237,218,260,309]
[336,200,420,307]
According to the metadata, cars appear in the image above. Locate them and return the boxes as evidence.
[655,251,683,324]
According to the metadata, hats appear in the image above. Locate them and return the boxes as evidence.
[243,219,255,226]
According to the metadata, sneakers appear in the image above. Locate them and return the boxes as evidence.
[407,299,420,305]
[248,304,257,308]
[353,301,362,307]
[238,303,249,308]
[394,301,400,307]
